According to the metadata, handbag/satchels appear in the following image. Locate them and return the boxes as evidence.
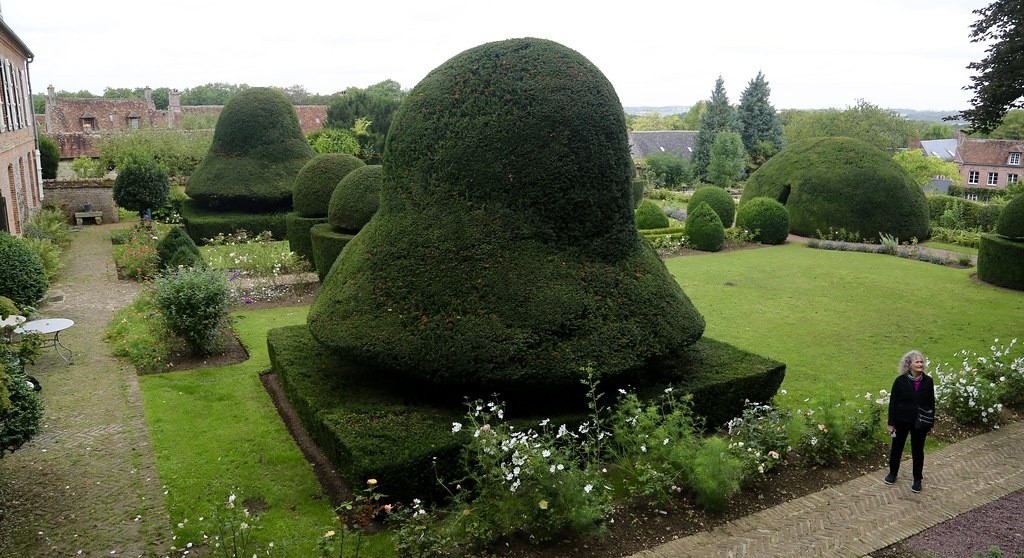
[913,406,934,431]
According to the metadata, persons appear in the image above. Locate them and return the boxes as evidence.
[884,350,935,493]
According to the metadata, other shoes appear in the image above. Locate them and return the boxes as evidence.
[910,479,922,493]
[883,472,898,485]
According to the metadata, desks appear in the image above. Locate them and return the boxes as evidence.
[14,318,74,368]
[0,314,27,345]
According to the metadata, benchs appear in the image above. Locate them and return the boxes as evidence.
[75,210,103,225]
